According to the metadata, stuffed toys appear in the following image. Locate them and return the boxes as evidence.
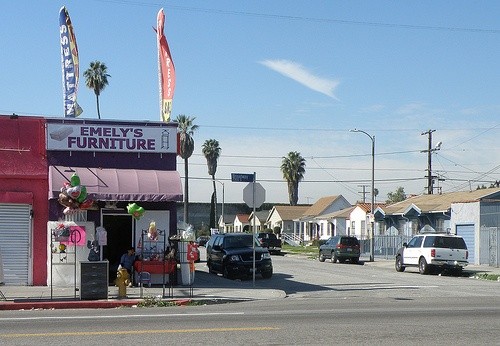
[148,222,159,240]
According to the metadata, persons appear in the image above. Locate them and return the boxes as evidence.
[121,247,136,287]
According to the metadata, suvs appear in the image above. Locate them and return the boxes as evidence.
[395,234,469,275]
[207,233,273,280]
[318,236,360,264]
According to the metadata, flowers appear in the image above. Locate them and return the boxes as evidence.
[54,224,70,237]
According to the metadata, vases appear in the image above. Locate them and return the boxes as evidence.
[55,236,69,241]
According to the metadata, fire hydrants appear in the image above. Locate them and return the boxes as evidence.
[118,266,130,299]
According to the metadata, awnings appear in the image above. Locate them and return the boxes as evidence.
[48,165,184,201]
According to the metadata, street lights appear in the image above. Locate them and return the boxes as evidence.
[349,128,375,260]
[209,180,224,234]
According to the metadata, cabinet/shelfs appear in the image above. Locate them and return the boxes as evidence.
[81,260,109,300]
[50,229,76,300]
[169,228,195,298]
[140,229,165,298]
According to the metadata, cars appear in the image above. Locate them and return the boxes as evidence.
[196,236,210,246]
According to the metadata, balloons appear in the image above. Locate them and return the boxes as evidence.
[58,172,96,215]
[126,202,144,221]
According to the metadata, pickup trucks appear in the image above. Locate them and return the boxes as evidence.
[255,233,281,254]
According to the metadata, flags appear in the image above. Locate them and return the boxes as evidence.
[59,5,83,117]
[156,8,176,122]
